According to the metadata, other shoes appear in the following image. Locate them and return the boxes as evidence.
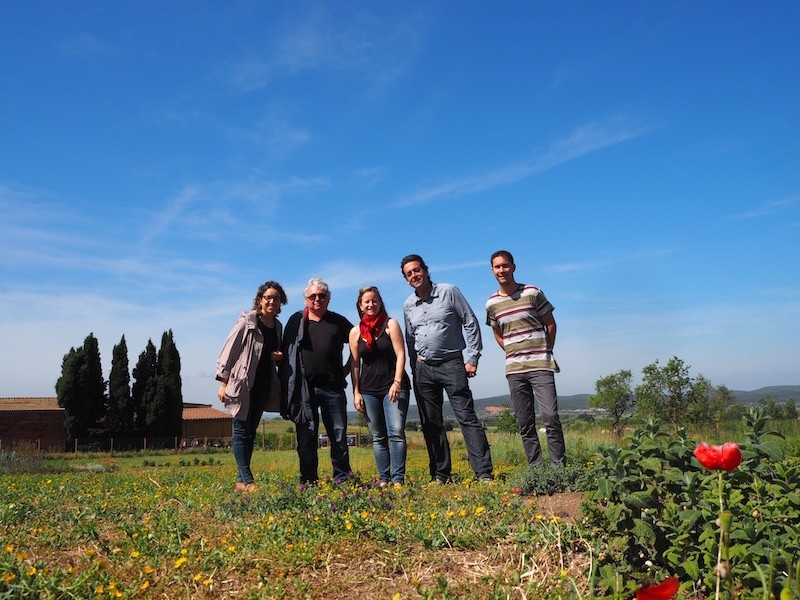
[394,482,402,487]
[477,476,492,482]
[236,483,256,491]
[428,478,444,485]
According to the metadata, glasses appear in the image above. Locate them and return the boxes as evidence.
[262,295,281,301]
[307,294,328,300]
[363,286,377,291]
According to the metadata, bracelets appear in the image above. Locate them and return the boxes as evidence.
[393,379,402,384]
[394,383,401,390]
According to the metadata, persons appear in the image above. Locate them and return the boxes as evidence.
[215,281,289,490]
[348,287,412,488]
[485,250,566,467]
[400,254,493,484]
[282,277,354,484]
